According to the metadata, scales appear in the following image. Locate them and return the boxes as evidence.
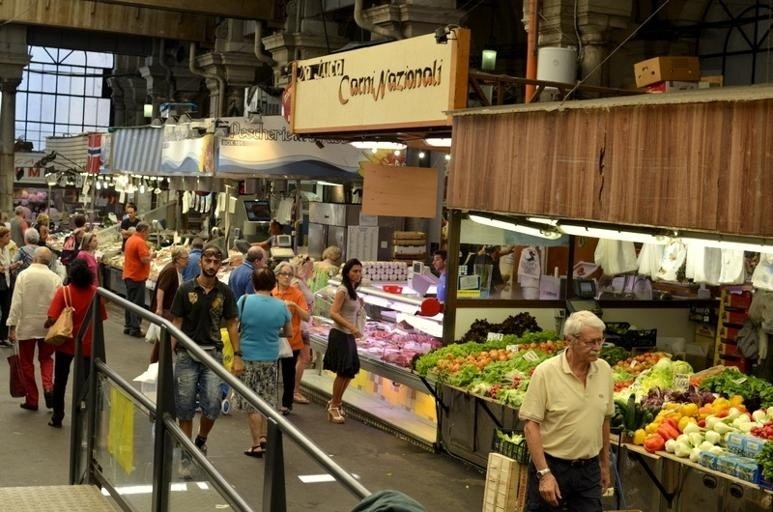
[270,234,295,261]
[566,279,632,351]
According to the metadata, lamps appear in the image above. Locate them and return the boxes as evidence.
[43,165,54,178]
[432,23,459,47]
[142,94,154,117]
[91,175,161,194]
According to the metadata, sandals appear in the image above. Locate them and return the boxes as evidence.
[278,393,310,415]
[244,436,267,458]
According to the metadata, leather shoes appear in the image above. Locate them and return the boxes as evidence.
[21,389,62,427]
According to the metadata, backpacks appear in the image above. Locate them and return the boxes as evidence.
[61,230,81,266]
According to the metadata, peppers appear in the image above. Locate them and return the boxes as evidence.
[633,394,751,453]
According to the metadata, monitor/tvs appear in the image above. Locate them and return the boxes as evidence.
[573,279,596,298]
[277,234,292,247]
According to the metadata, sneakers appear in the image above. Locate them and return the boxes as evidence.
[124,328,146,337]
[179,445,208,478]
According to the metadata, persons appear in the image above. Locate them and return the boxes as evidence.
[305,245,341,318]
[120,205,142,254]
[475,244,514,293]
[2,206,108,428]
[122,222,156,337]
[734,288,773,385]
[430,247,446,308]
[516,309,614,510]
[320,257,367,423]
[149,220,309,466]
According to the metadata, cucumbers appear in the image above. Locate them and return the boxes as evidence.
[610,393,661,434]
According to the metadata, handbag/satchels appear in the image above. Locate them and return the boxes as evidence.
[220,328,245,376]
[278,337,294,359]
[7,339,29,397]
[43,307,76,347]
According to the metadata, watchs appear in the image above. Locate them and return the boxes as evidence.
[535,466,551,480]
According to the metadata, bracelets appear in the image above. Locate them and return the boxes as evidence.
[234,351,243,357]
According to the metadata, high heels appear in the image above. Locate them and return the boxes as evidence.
[327,399,347,424]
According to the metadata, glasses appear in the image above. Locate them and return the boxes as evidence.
[201,250,222,259]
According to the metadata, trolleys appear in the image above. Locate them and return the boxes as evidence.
[191,375,230,417]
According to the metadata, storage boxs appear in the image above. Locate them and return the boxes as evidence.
[632,55,701,93]
[480,452,528,511]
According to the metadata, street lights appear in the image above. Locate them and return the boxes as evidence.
[43,172,58,233]
